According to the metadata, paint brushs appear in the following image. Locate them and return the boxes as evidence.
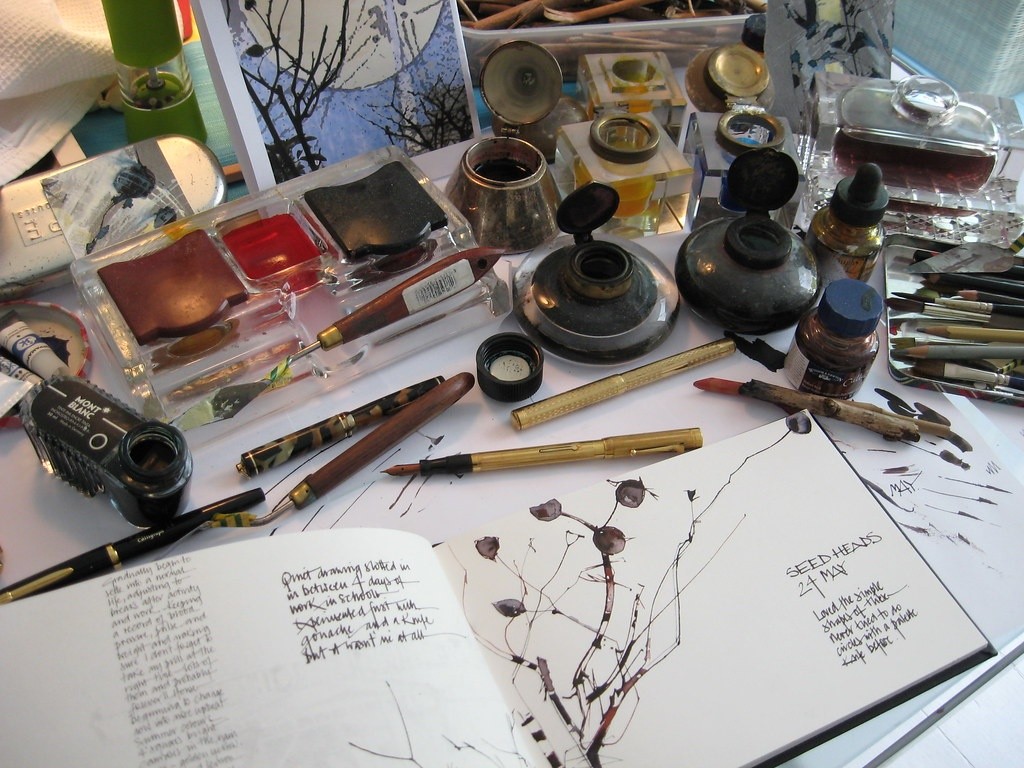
[890,291,1024,319]
[913,359,1024,391]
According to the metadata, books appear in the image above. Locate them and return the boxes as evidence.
[0,406,994,768]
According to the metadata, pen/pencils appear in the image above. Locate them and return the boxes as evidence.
[0,487,267,608]
[237,375,449,478]
[885,250,1024,393]
[511,336,738,430]
[378,425,702,477]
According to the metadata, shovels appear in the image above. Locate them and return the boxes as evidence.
[906,232,1024,274]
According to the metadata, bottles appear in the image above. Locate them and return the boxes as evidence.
[782,279,881,401]
[806,162,890,288]
